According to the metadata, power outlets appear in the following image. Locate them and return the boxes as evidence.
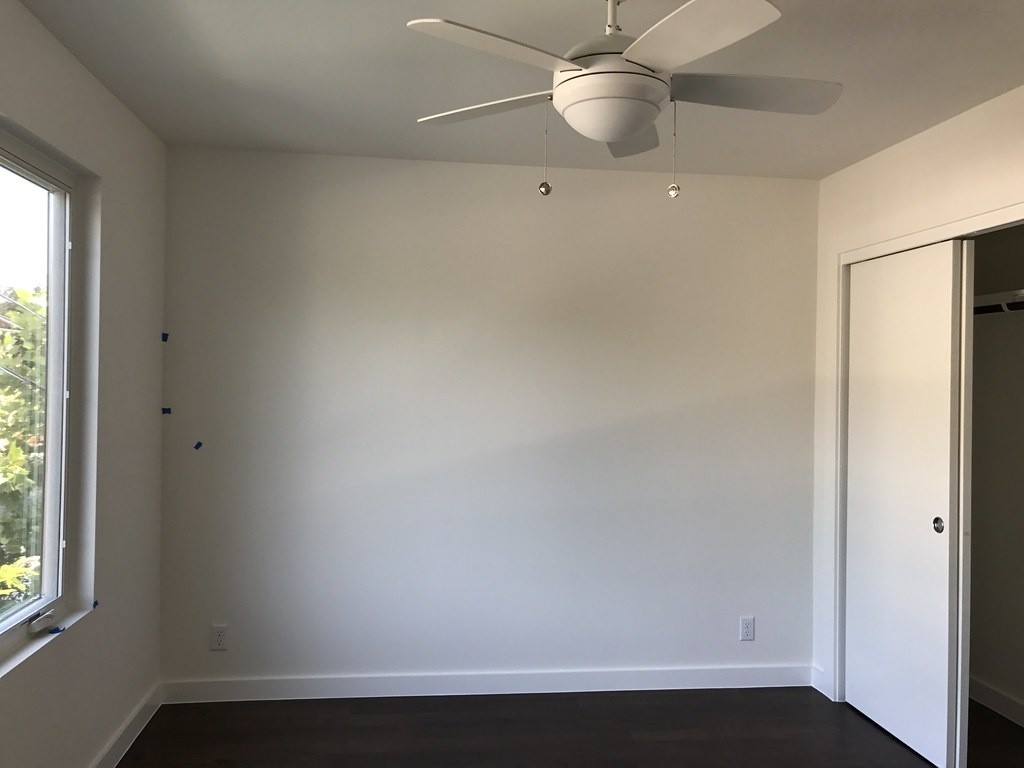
[209,624,229,650]
[740,616,755,642]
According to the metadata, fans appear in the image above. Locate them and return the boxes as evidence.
[406,0,845,199]
